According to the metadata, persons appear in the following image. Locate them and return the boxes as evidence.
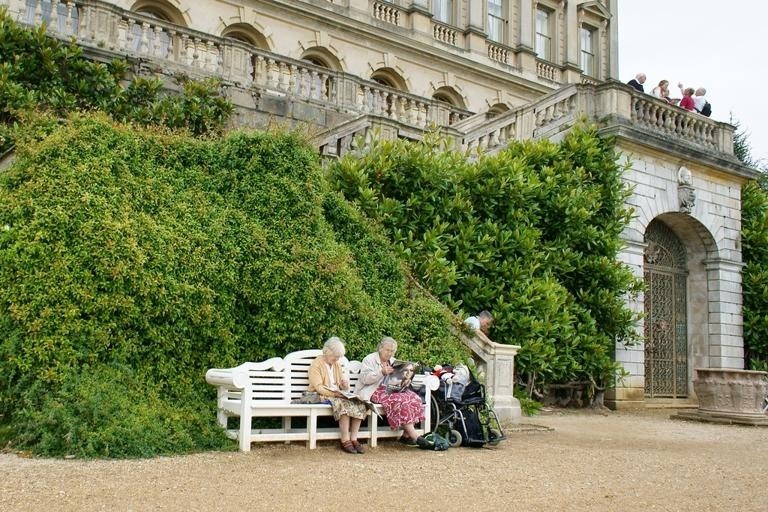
[290,337,367,454]
[354,336,435,448]
[390,363,415,393]
[628,72,706,115]
[463,310,494,331]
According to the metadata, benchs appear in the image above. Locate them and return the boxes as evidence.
[205,348,441,453]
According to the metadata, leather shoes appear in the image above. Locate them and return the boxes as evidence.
[340,439,358,453]
[416,435,431,446]
[399,435,416,446]
[351,440,365,454]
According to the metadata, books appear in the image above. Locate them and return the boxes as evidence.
[324,386,384,421]
[381,358,421,393]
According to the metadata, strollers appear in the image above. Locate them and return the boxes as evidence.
[422,364,507,447]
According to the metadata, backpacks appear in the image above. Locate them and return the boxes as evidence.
[454,407,485,448]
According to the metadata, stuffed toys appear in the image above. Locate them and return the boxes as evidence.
[433,364,460,384]
